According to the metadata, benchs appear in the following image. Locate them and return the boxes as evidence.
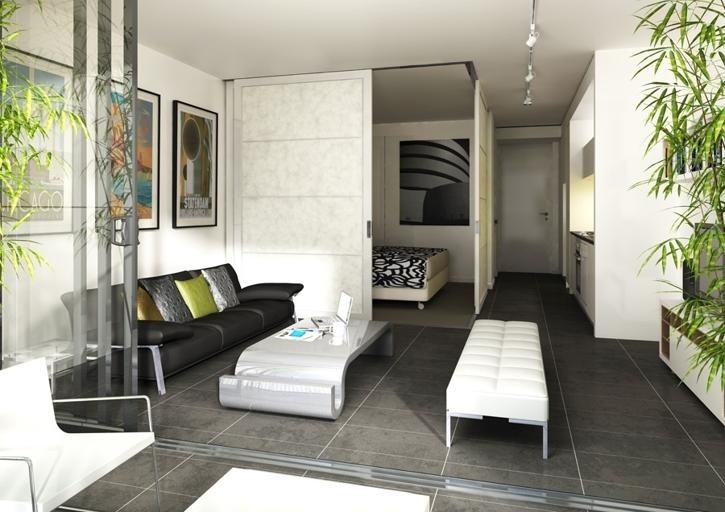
[443,318,551,460]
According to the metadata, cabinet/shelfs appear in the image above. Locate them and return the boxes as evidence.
[567,233,595,326]
[657,302,725,428]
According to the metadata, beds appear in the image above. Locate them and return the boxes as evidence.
[372,246,450,310]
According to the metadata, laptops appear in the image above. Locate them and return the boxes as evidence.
[311,290,354,332]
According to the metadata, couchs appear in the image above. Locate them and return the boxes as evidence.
[62,262,304,397]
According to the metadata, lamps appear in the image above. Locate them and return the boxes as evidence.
[524,14,539,106]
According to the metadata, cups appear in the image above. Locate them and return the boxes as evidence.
[329,337,343,346]
[329,322,346,337]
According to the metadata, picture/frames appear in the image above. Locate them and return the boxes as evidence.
[0,44,73,237]
[95,75,161,230]
[172,100,218,229]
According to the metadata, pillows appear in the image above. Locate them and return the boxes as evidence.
[119,265,241,329]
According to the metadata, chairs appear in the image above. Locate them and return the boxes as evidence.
[0,355,163,512]
